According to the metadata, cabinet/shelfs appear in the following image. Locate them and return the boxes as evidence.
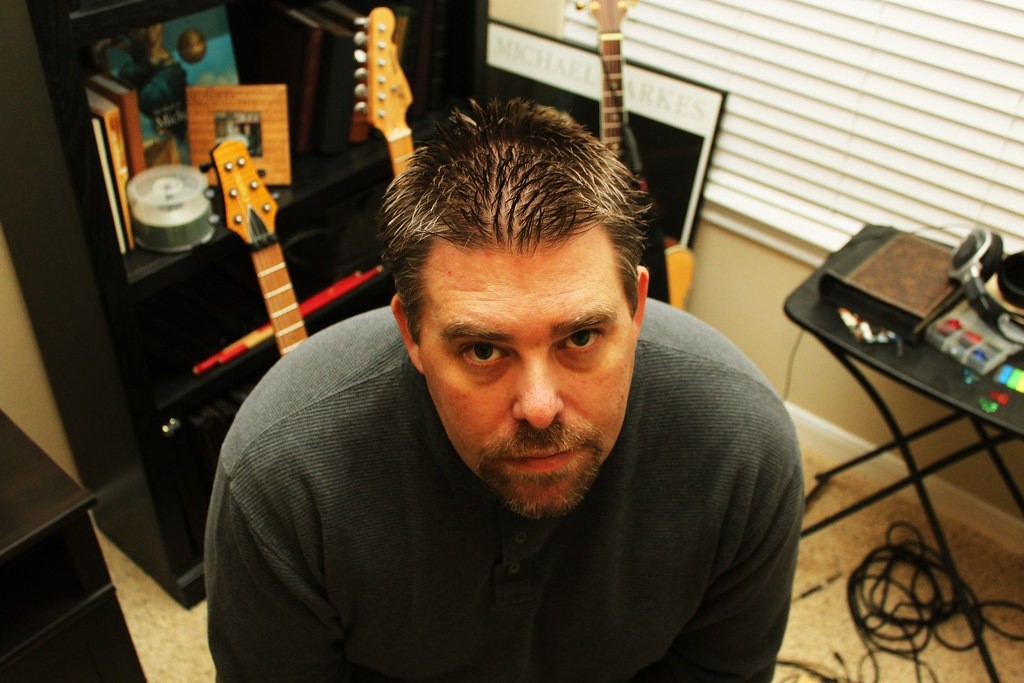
[0,408,149,683]
[0,1,489,610]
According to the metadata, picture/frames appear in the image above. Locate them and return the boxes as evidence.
[486,16,729,249]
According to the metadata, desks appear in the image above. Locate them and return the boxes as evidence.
[784,223,1024,683]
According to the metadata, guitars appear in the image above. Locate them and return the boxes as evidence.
[574,2,698,310]
[195,128,310,360]
[351,6,420,182]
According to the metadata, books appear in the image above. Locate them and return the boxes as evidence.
[818,224,967,346]
[87,69,147,254]
[283,2,407,153]
[203,91,806,682]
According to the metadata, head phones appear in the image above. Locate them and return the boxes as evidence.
[948,227,1024,326]
